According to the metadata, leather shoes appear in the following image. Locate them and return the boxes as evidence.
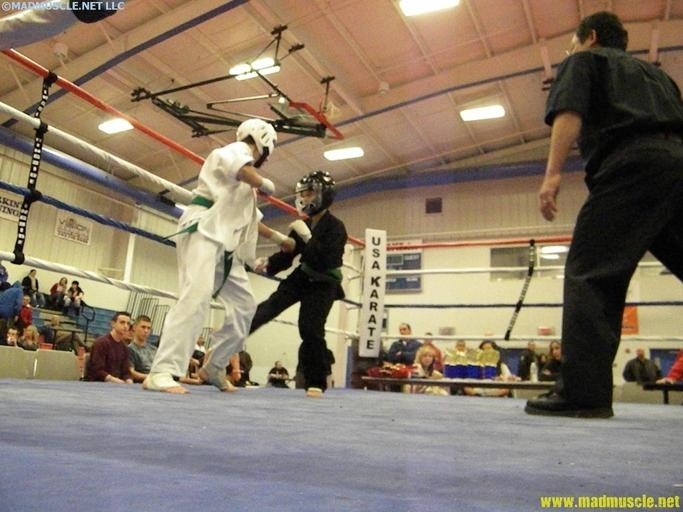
[525,391,613,419]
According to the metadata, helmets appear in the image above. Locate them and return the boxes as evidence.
[295,170,336,217]
[237,118,277,167]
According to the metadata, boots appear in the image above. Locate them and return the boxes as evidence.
[63,306,68,315]
[75,308,79,316]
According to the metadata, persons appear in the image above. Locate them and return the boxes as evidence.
[268,359,287,386]
[524,12,682,419]
[139,117,295,393]
[348,327,561,394]
[325,340,333,381]
[83,307,252,385]
[0,261,83,351]
[622,346,662,382]
[292,365,304,388]
[655,350,682,384]
[245,169,346,397]
